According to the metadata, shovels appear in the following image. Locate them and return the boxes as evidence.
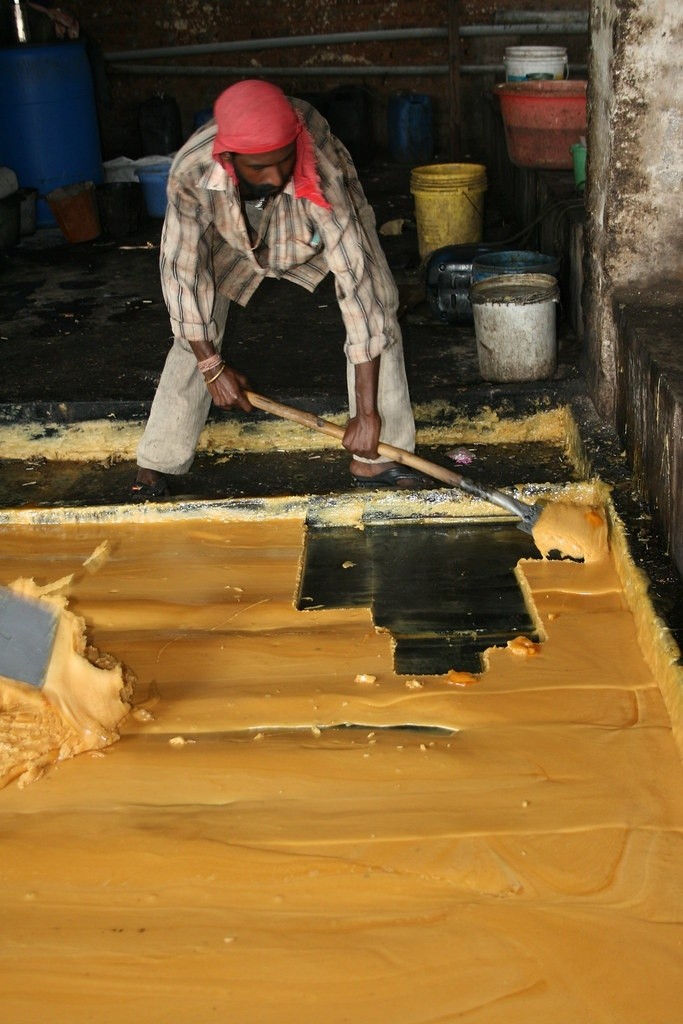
[236,390,549,537]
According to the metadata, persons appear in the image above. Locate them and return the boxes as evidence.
[130,80,436,500]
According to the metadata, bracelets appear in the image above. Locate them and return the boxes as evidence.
[198,353,225,383]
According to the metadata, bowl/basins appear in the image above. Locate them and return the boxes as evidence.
[496,81,588,167]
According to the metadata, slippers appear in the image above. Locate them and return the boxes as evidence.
[128,477,170,501]
[354,465,437,490]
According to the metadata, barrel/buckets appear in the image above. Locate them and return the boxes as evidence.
[0,194,20,250]
[135,163,172,219]
[570,145,590,192]
[410,162,489,260]
[96,182,142,238]
[387,93,433,161]
[44,182,102,245]
[502,45,570,82]
[18,186,39,237]
[467,273,561,383]
[472,251,561,282]
